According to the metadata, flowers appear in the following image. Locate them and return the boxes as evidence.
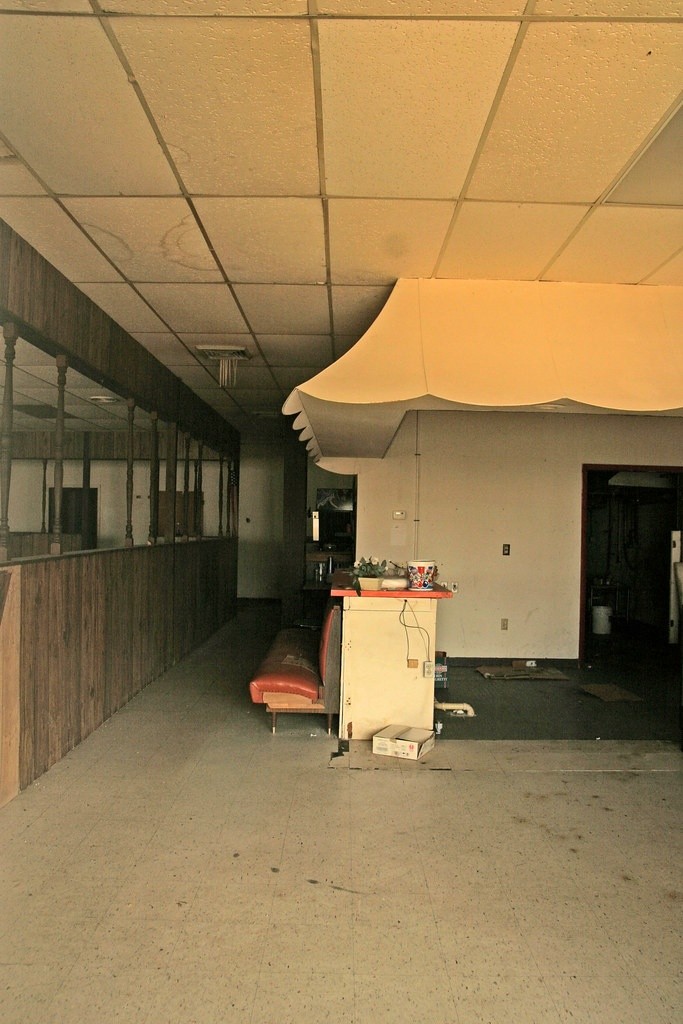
[350,555,387,591]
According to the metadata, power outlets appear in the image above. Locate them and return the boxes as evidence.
[424,661,436,678]
[500,619,508,630]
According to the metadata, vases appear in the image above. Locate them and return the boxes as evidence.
[357,576,384,592]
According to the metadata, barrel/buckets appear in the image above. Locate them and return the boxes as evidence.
[592,606,612,634]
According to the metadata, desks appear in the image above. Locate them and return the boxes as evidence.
[586,583,634,626]
[307,551,353,582]
[301,580,332,618]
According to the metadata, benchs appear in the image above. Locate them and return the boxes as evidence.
[250,596,342,734]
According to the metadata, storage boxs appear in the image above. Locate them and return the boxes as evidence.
[373,724,436,760]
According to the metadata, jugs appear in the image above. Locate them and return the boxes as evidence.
[326,556,334,574]
